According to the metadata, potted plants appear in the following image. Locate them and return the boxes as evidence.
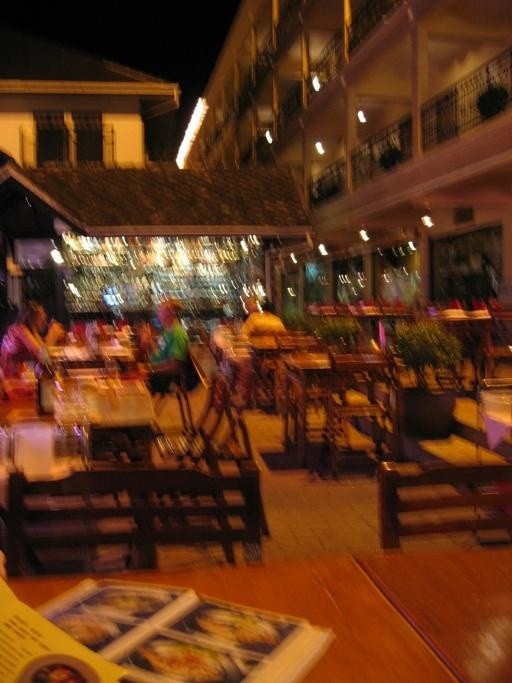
[389,321,463,438]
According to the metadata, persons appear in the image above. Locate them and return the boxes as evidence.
[136,300,201,398]
[237,297,261,323]
[0,301,67,363]
[240,301,286,336]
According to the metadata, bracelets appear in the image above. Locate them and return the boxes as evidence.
[147,361,158,375]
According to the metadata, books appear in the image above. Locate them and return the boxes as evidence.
[0,576,133,683]
[32,577,338,683]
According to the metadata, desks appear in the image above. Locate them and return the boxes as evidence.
[0,547,512,682]
[46,344,136,373]
[282,349,380,464]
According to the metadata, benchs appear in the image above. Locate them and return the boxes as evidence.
[155,371,254,476]
[0,460,261,574]
[478,312,512,390]
[254,333,322,411]
[54,373,155,468]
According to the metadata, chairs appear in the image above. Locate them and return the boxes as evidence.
[321,349,387,477]
[274,330,350,432]
